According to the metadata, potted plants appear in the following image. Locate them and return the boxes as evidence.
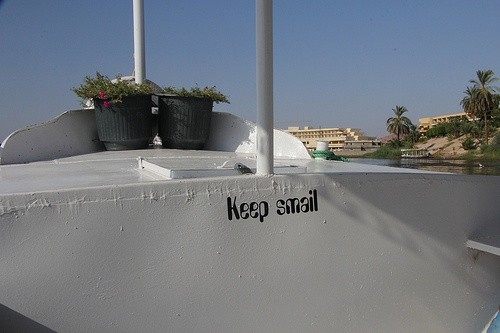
[151,84,231,150]
[71,71,154,151]
